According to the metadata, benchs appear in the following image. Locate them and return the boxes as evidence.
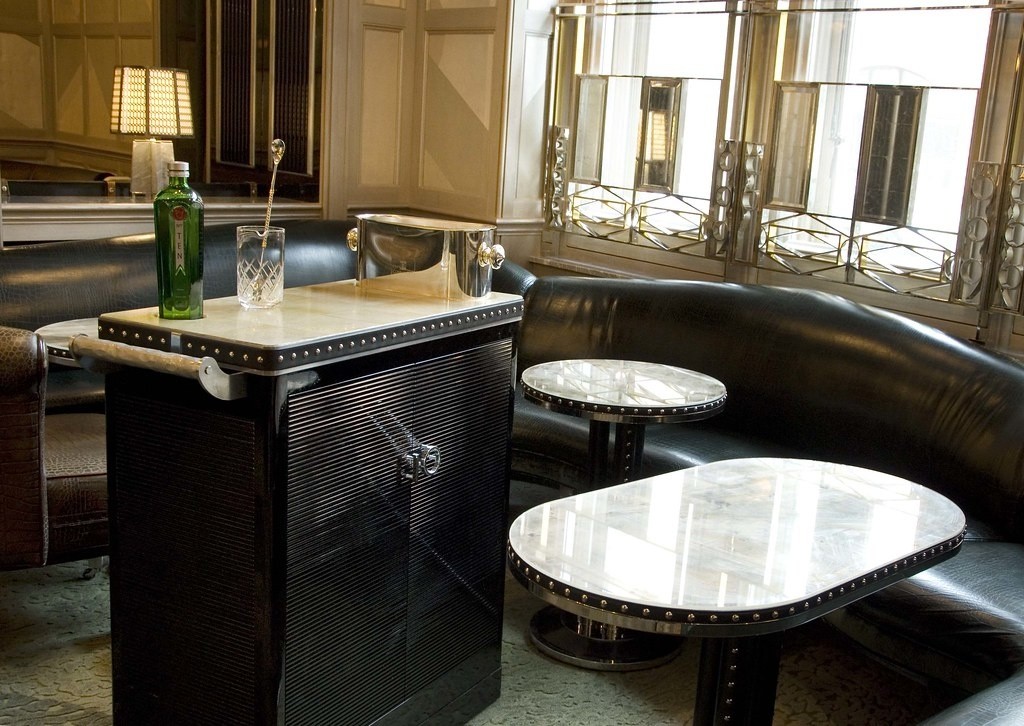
[0,218,1024,726]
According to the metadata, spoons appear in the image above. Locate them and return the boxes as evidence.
[252,139,285,300]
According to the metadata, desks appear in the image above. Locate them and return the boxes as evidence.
[517,358,731,671]
[509,457,969,726]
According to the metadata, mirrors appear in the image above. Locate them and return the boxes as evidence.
[0,0,329,214]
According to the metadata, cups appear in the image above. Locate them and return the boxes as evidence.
[236,225,284,310]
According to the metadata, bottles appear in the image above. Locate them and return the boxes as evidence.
[153,161,204,320]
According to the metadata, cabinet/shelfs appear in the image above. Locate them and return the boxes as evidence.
[69,272,525,726]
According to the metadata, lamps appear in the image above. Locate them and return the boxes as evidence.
[108,66,197,195]
